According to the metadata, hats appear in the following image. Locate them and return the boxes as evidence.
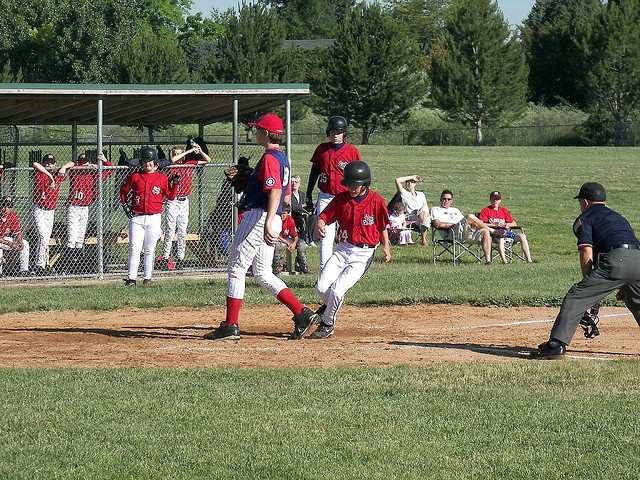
[248,114,284,134]
[78,154,91,164]
[1,196,14,208]
[573,182,606,202]
[490,191,501,199]
[43,154,55,163]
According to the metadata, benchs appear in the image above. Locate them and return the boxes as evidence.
[45,233,202,271]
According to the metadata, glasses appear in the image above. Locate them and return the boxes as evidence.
[257,129,266,134]
[443,198,451,200]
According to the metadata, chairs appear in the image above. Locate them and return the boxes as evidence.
[388,219,416,246]
[476,211,526,263]
[431,222,482,265]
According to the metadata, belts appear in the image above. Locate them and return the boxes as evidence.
[607,243,640,253]
[177,197,186,201]
[349,242,375,248]
[79,204,86,207]
[38,206,53,210]
[133,213,153,215]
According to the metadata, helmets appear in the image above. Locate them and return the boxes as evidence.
[326,116,347,137]
[340,161,371,188]
[139,148,157,165]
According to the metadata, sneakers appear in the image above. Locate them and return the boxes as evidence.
[304,241,311,247]
[143,279,153,284]
[314,303,327,324]
[308,241,318,247]
[125,280,136,286]
[204,321,240,340]
[159,259,168,271]
[289,307,319,340]
[531,338,566,359]
[175,259,184,270]
[311,321,334,339]
[491,229,507,238]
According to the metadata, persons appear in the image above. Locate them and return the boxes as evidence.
[218,152,252,209]
[388,201,416,246]
[226,200,245,234]
[479,190,534,265]
[162,144,212,273]
[271,202,312,276]
[292,174,318,248]
[530,181,640,358]
[29,153,75,276]
[0,160,14,181]
[309,160,392,339]
[430,189,508,266]
[201,113,321,341]
[395,173,431,247]
[120,147,183,287]
[63,150,118,264]
[1,195,32,277]
[305,114,362,287]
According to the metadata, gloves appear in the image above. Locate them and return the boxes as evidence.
[121,203,135,219]
[172,172,180,185]
[1,162,16,169]
[190,139,197,147]
